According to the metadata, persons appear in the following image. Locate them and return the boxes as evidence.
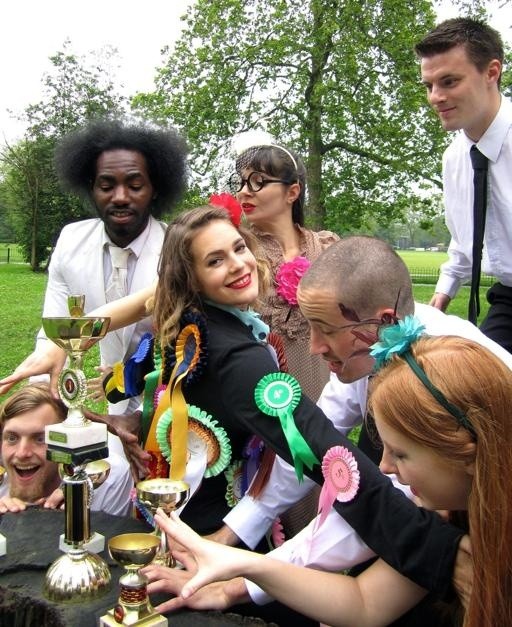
[414,15,511,353]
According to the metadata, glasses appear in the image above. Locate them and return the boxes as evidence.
[226,171,293,192]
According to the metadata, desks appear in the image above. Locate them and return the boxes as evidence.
[0,505,321,627]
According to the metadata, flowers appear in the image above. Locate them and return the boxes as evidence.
[334,285,427,373]
[210,192,241,227]
[275,256,312,306]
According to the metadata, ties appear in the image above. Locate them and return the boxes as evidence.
[468,145,490,328]
[105,245,131,306]
